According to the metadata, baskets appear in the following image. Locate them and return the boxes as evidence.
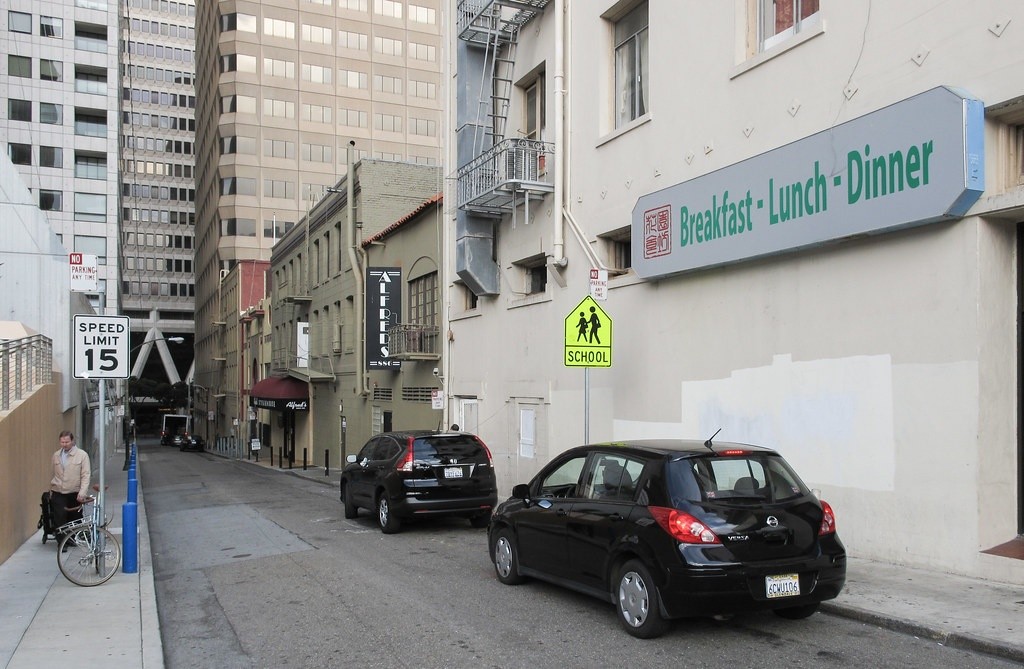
[56,516,93,535]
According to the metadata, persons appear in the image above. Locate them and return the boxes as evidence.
[50,430,91,552]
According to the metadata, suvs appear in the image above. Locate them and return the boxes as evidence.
[338,429,498,535]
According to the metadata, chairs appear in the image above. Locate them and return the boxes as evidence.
[598,465,637,501]
[733,477,759,491]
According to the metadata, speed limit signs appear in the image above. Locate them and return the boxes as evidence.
[72,312,133,380]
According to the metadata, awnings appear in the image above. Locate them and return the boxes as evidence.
[250,378,309,412]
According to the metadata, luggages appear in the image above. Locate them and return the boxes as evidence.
[42,490,56,544]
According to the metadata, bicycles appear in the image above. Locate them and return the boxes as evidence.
[54,484,122,586]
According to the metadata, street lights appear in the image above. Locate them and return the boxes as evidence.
[192,384,209,450]
[123,337,184,471]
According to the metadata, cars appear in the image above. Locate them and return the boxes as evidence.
[487,436,847,640]
[160,432,184,447]
[182,434,205,453]
